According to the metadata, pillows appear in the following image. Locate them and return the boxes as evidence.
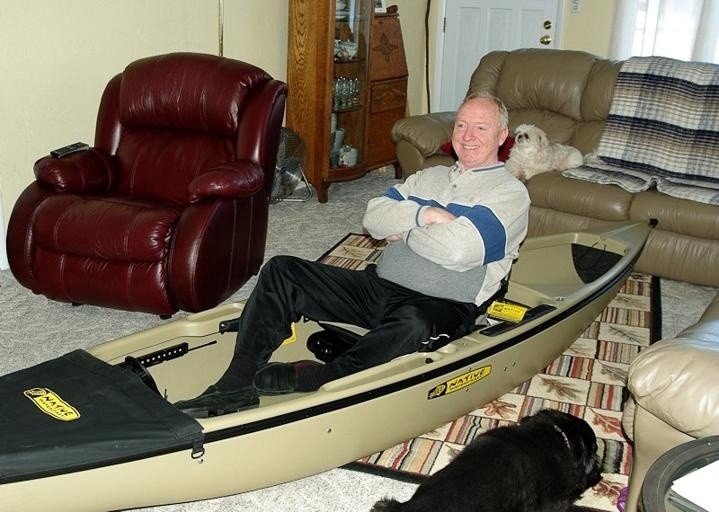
[441,131,519,168]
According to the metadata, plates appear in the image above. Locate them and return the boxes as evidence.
[335,15,346,19]
[335,11,350,16]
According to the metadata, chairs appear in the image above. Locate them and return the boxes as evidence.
[4,51,289,321]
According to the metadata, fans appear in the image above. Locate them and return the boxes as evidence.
[268,126,312,206]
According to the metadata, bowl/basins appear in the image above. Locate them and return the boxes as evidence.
[335,2,347,11]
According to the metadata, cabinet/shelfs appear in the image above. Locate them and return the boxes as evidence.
[286,0,412,202]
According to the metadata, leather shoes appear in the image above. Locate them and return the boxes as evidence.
[253,360,327,395]
[174,382,260,418]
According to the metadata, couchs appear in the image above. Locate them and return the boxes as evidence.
[389,45,719,292]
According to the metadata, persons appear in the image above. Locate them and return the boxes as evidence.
[171,89,533,419]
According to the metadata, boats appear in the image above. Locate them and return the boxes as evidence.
[0,213,660,511]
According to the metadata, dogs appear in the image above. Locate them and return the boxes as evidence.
[368,407,605,512]
[503,122,585,185]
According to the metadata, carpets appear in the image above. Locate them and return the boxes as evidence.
[336,273,667,510]
